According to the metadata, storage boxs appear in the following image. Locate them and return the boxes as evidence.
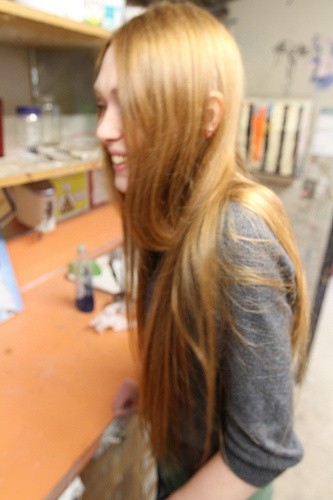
[59,112,99,150]
[0,187,19,232]
[238,99,316,182]
[89,167,112,209]
[50,170,90,224]
[4,178,57,235]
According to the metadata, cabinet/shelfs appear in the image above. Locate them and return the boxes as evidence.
[0,0,111,186]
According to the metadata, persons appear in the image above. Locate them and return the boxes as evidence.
[92,1,312,500]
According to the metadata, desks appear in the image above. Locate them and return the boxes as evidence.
[0,196,143,500]
[246,161,333,386]
